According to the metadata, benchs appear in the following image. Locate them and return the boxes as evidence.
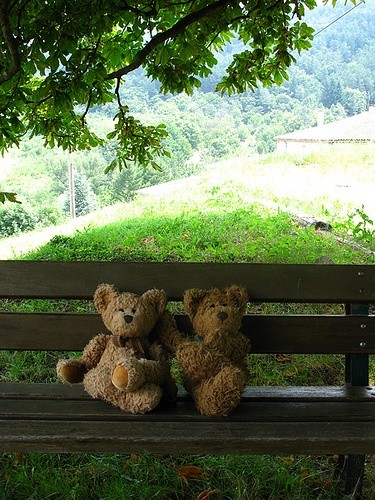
[1,260,375,500]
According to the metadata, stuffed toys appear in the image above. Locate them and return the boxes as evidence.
[158,282,251,418]
[56,283,168,412]
